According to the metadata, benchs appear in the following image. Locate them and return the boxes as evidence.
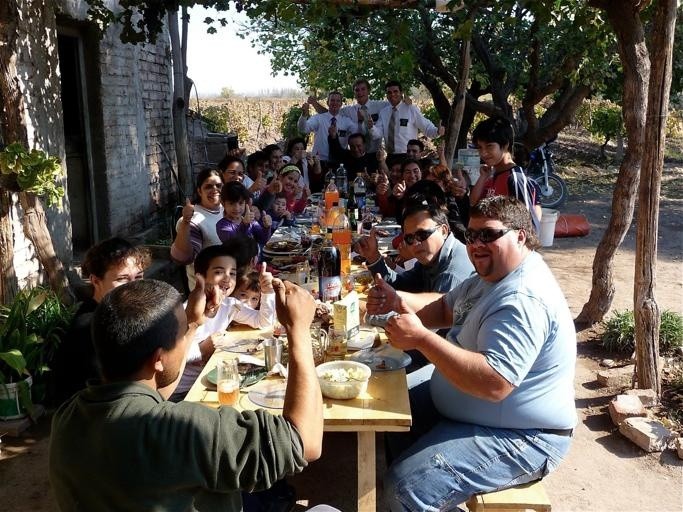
[468,481,554,512]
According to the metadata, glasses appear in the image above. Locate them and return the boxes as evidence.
[404,228,435,245]
[464,227,512,243]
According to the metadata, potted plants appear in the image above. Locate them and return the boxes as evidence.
[0,281,52,429]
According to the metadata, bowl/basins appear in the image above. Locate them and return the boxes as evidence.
[315,360,372,401]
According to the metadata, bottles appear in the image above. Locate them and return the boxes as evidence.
[318,165,367,304]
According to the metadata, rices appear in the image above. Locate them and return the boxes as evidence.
[319,368,365,399]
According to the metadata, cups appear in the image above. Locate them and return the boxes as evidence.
[294,261,311,286]
[214,358,239,404]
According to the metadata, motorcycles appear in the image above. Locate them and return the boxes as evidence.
[467,108,567,209]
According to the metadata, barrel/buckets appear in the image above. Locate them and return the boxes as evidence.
[537,208,560,248]
[459,148,481,168]
[0,375,33,421]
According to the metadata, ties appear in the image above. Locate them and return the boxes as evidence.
[388,107,397,153]
[361,106,370,152]
[329,117,336,159]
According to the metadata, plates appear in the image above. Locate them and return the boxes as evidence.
[262,202,316,275]
[261,323,346,369]
[205,362,265,388]
[368,200,402,239]
[220,338,260,353]
[351,347,412,372]
[246,378,287,409]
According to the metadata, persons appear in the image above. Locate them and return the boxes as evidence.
[365,194,579,511]
[48,278,323,512]
[48,77,530,416]
